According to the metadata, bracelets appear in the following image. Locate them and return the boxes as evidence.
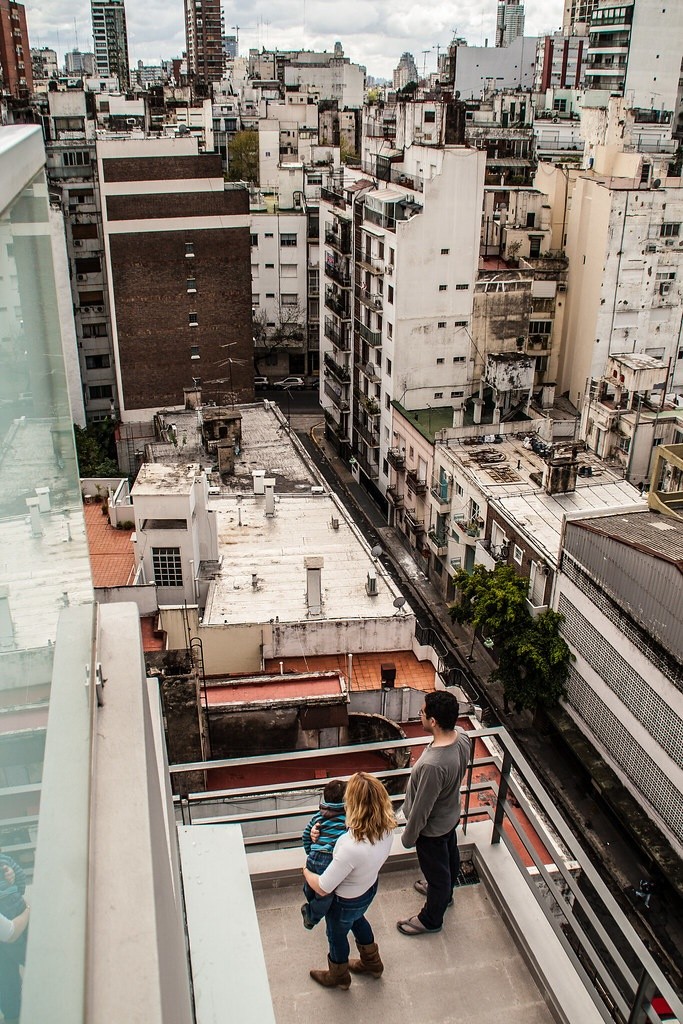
[302,868,307,873]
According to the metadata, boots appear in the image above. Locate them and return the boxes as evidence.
[349,940,384,980]
[310,953,351,991]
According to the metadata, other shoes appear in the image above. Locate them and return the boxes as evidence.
[301,903,315,931]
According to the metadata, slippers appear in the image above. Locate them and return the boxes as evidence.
[414,879,454,907]
[398,914,445,936]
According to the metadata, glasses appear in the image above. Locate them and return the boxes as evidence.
[418,709,426,717]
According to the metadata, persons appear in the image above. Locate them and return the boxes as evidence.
[396,690,470,935]
[0,852,30,1024]
[301,772,397,991]
